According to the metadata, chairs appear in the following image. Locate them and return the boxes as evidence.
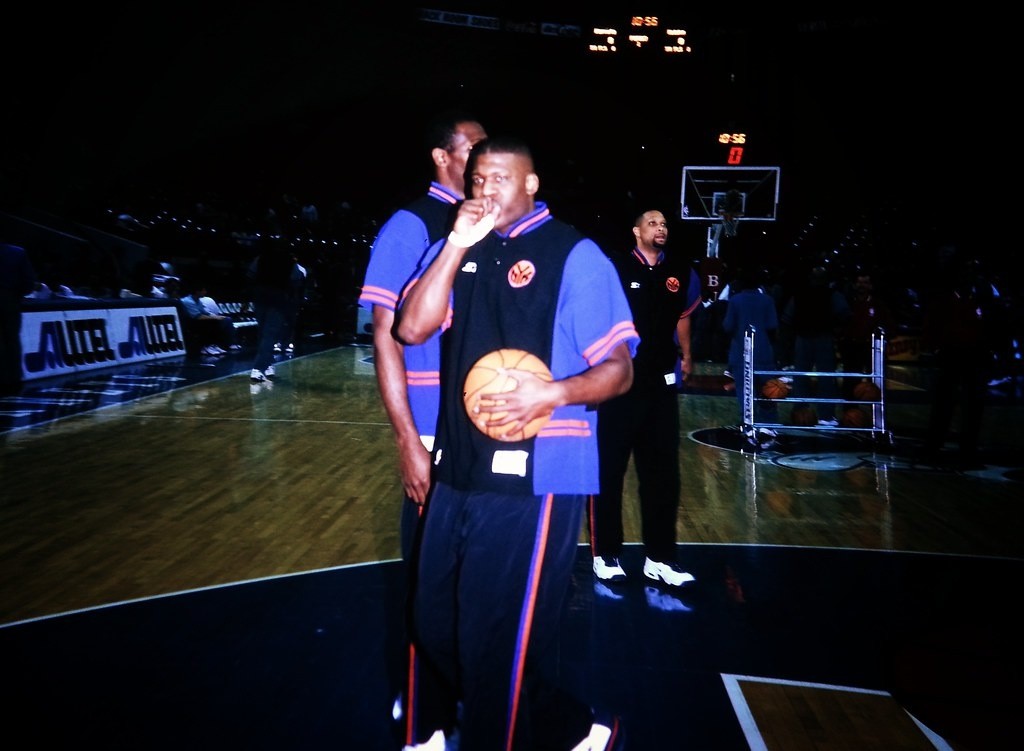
[200,294,261,328]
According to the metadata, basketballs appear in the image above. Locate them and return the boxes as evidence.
[764,380,786,400]
[464,348,555,443]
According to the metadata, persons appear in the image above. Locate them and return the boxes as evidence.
[178,280,242,355]
[359,117,638,751]
[584,210,702,590]
[717,256,890,425]
[247,236,307,381]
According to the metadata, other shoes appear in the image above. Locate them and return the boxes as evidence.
[250,383,262,394]
[818,419,840,426]
[745,430,753,436]
[250,368,266,381]
[571,723,612,751]
[760,428,778,437]
[761,438,775,449]
[264,365,275,376]
[401,727,461,751]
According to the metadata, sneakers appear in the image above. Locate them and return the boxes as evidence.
[592,554,628,583]
[643,555,699,591]
